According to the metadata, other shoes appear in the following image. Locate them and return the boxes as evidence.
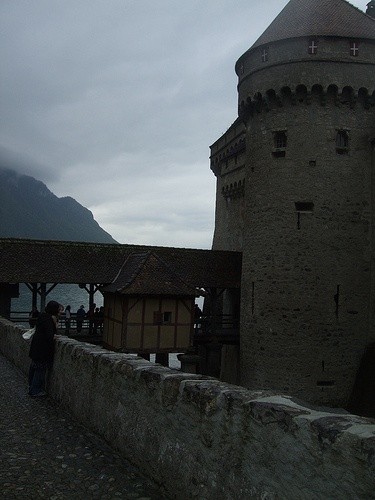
[33,391,48,396]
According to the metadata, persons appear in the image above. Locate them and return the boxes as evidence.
[76,305,86,332]
[64,305,72,329]
[27,301,64,397]
[29,306,39,329]
[92,303,105,336]
[194,304,202,329]
